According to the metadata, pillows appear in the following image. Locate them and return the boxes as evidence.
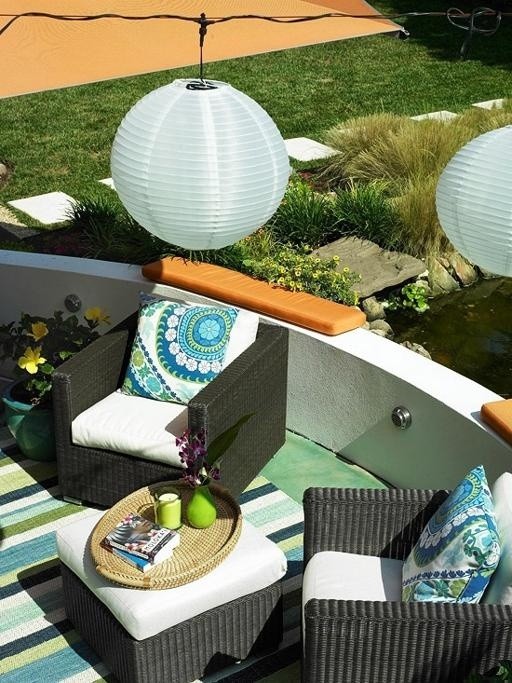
[118,288,258,403]
[397,460,503,606]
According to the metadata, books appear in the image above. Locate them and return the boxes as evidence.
[99,512,180,574]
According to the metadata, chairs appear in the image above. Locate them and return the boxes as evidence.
[295,463,510,681]
[42,302,289,512]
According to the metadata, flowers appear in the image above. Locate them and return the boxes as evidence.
[175,425,224,487]
[0,288,109,399]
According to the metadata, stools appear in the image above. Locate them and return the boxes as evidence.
[54,493,287,681]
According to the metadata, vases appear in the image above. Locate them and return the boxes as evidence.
[0,376,56,461]
[187,476,217,529]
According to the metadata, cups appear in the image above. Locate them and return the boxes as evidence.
[152,484,184,531]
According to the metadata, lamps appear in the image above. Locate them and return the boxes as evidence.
[436,120,511,284]
[107,13,290,250]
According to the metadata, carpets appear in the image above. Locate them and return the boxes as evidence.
[0,415,305,682]
